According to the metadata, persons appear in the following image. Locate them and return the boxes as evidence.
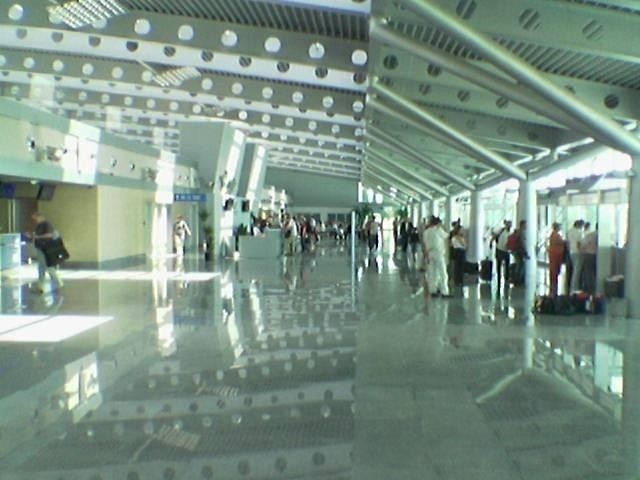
[549,220,599,301]
[421,214,467,298]
[171,254,191,301]
[23,211,66,294]
[365,215,382,250]
[490,220,529,299]
[281,213,323,255]
[393,218,419,251]
[332,224,351,241]
[173,213,192,260]
[251,211,279,236]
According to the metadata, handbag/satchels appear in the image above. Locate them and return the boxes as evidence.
[535,289,607,316]
[42,237,70,268]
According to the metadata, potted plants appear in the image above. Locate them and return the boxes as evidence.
[348,203,374,241]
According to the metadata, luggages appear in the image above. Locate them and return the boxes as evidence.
[480,258,493,282]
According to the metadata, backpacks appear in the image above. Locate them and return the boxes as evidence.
[507,233,519,250]
